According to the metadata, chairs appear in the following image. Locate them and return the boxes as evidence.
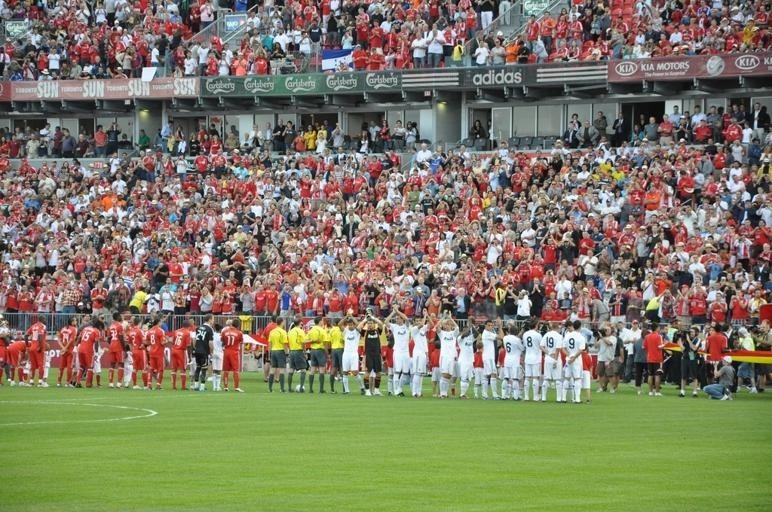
[341,141,349,150]
[350,138,361,150]
[462,135,559,151]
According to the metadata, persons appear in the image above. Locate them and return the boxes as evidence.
[0,103,771,404]
[0,0,771,81]
